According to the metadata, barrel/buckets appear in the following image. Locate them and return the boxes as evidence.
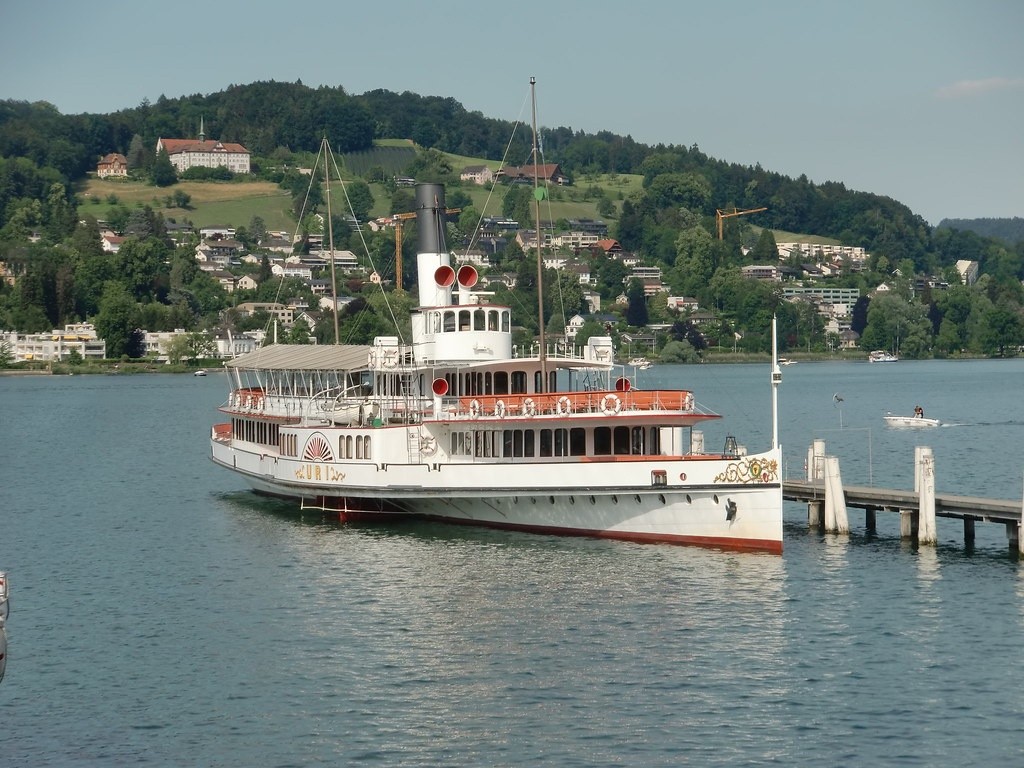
[373,419,381,427]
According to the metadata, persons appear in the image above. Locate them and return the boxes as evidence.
[915,406,923,419]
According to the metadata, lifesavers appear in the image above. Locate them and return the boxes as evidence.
[522,398,535,418]
[495,400,505,420]
[601,394,622,416]
[470,400,479,420]
[558,396,571,417]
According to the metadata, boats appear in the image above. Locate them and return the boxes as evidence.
[629,357,650,366]
[206,76,783,557]
[639,363,652,370]
[882,414,942,428]
[869,350,898,363]
[194,370,206,377]
